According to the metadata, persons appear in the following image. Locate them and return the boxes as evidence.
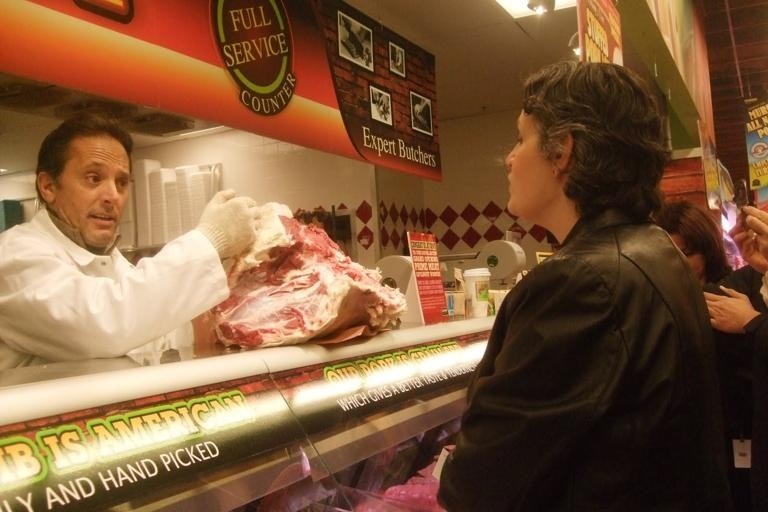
[0,113,262,361]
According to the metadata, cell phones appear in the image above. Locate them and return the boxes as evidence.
[734,179,751,232]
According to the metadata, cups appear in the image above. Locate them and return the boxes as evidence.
[463,266,491,319]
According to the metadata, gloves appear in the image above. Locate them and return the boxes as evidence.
[198,190,262,260]
